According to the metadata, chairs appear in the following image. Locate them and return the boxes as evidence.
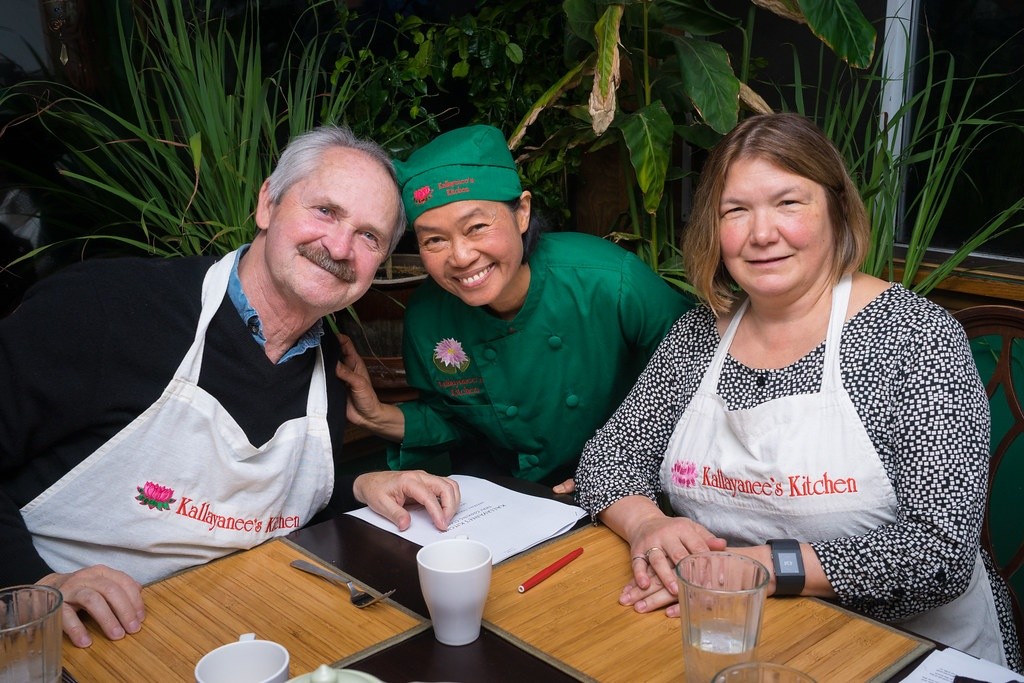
[952,305,1024,672]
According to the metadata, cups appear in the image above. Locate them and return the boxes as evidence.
[712,661,817,683]
[0,585,64,683]
[416,534,493,646]
[676,551,770,683]
[285,664,385,683]
[194,632,290,683]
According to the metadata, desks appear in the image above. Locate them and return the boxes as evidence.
[0,481,1024,683]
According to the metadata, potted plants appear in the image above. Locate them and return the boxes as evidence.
[0,0,590,526]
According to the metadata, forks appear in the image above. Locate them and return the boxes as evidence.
[290,560,397,609]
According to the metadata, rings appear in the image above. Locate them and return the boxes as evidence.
[646,547,663,556]
[633,557,649,566]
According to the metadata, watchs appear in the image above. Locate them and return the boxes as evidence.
[766,539,805,595]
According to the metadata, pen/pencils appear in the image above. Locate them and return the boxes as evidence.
[518,547,584,594]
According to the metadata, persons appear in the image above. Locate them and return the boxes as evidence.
[336,125,694,493]
[0,129,461,648]
[572,112,1024,676]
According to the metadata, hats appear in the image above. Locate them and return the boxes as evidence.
[391,125,521,227]
